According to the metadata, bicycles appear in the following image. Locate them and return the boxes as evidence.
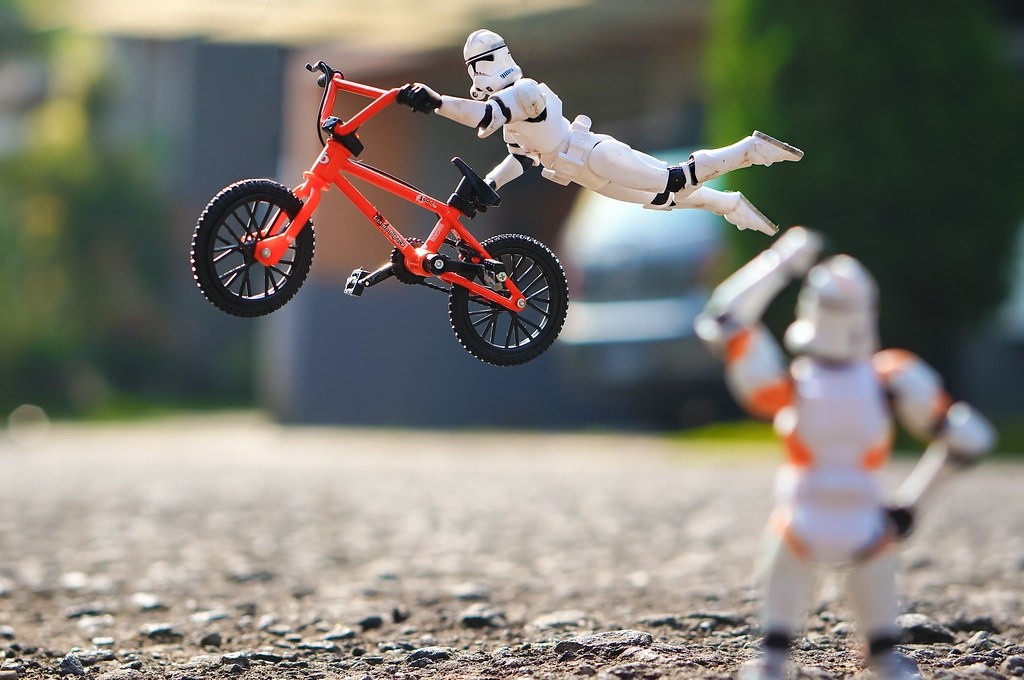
[189,59,570,367]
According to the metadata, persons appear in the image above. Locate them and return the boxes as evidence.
[395,28,805,238]
[694,224,998,680]
